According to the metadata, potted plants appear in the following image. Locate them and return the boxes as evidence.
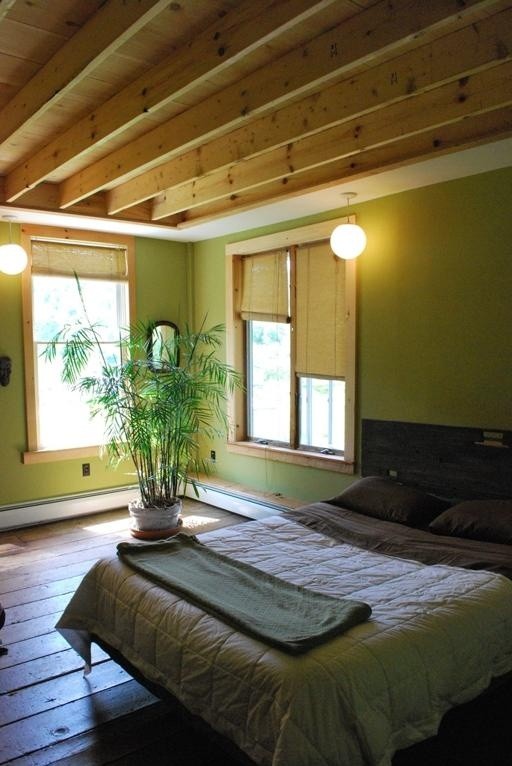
[43,272,247,530]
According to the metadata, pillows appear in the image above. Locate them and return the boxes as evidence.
[320,474,451,531]
[429,500,511,546]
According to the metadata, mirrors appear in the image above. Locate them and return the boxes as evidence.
[146,320,181,374]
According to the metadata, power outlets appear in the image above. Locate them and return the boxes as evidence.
[82,464,90,476]
[211,451,215,460]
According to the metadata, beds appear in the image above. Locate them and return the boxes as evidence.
[86,418,512,766]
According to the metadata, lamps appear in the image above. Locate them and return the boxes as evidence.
[0,215,29,276]
[330,191,367,259]
